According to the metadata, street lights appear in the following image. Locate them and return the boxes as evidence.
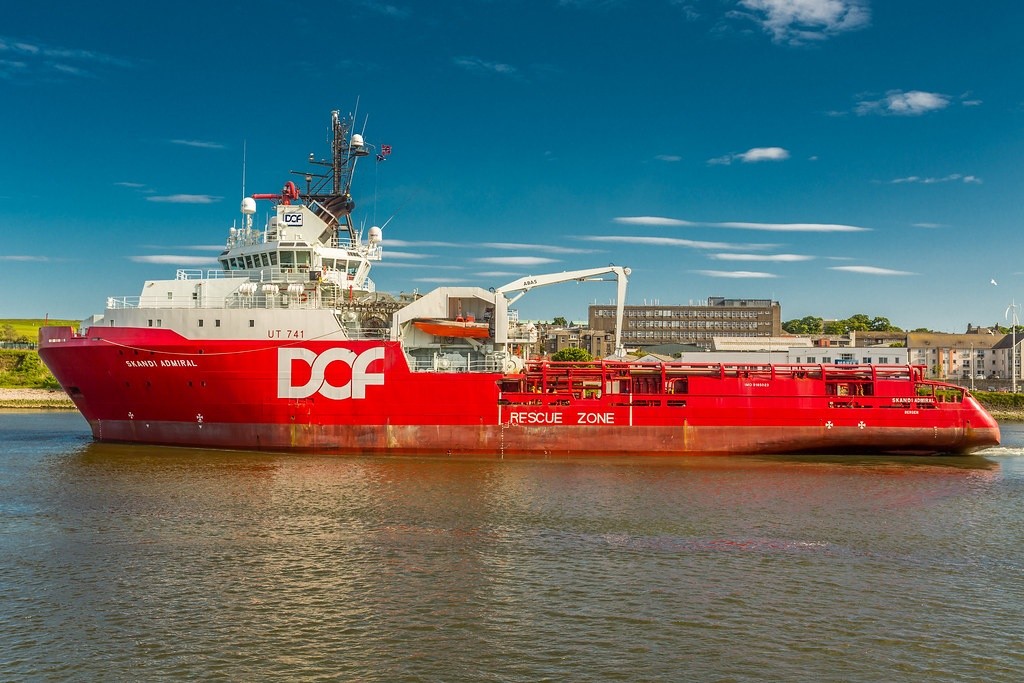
[970,343,973,391]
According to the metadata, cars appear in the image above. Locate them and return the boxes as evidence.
[987,374,1000,379]
[969,386,977,391]
[975,374,986,379]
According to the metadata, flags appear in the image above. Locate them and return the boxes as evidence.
[382,144,391,155]
[376,154,387,161]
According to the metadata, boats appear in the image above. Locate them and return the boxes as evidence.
[38,109,1001,455]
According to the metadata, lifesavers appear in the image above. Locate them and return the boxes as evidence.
[299,294,308,302]
